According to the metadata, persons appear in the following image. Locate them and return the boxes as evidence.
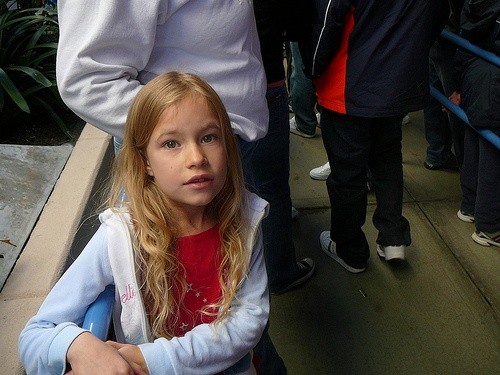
[18,72,270,375]
[252,0,500,295]
[57,0,288,375]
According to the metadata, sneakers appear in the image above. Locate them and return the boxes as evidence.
[292,207,299,219]
[271,257,315,295]
[456,209,475,223]
[376,244,406,263]
[309,161,331,180]
[319,230,366,274]
[471,230,500,246]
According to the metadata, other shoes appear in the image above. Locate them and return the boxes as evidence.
[402,115,411,125]
[289,116,316,138]
[316,113,321,127]
[424,157,460,172]
